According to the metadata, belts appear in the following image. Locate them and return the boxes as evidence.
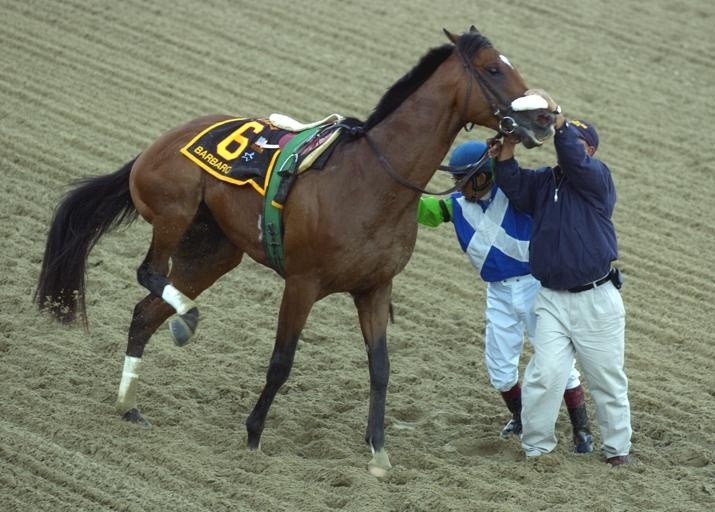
[563,273,617,294]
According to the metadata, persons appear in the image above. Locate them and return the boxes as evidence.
[496,88,646,474]
[418,141,596,454]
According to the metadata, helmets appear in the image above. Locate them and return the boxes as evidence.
[448,143,496,172]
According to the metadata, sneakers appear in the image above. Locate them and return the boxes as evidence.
[572,430,596,456]
[499,416,522,441]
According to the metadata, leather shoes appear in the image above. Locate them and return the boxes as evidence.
[606,450,628,472]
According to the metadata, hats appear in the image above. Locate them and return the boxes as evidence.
[561,118,598,149]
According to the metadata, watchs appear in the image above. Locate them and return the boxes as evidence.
[553,105,561,114]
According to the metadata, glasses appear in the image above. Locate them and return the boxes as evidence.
[450,175,483,184]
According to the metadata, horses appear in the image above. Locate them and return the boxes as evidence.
[32,23,558,479]
